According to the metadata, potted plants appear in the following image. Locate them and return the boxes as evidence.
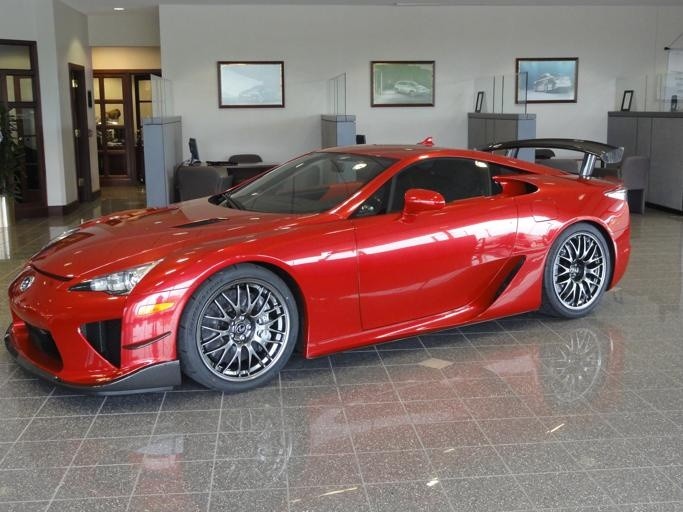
[0,98,32,261]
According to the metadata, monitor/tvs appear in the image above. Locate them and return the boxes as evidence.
[189,138,200,166]
[357,135,366,144]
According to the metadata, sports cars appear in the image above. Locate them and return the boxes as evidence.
[4,135,631,394]
[111,318,624,512]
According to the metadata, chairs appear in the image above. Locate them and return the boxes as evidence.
[534,146,650,215]
[174,152,281,202]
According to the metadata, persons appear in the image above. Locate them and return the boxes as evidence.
[95,108,123,174]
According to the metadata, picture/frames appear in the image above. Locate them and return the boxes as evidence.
[368,58,436,110]
[214,58,286,110]
[619,89,633,111]
[513,56,579,104]
